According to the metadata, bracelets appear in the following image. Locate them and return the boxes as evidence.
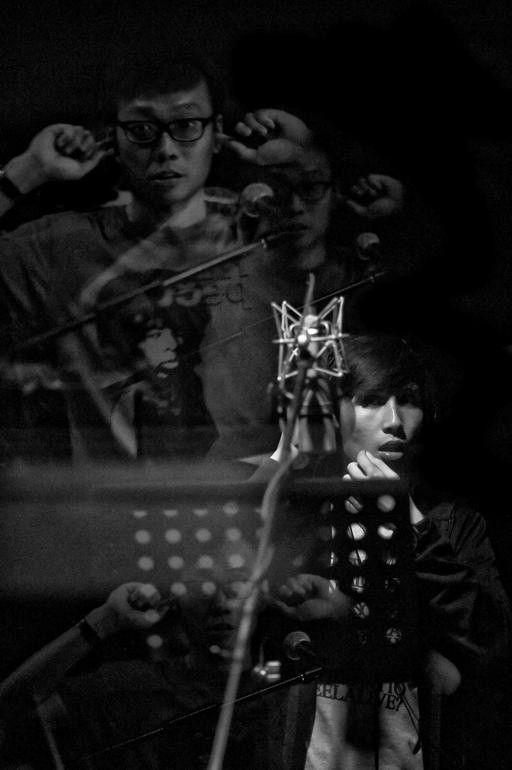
[0,168,25,205]
[409,518,431,554]
[76,616,104,650]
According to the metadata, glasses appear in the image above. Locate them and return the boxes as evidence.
[116,114,214,144]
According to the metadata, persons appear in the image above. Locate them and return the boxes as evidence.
[104,302,203,470]
[267,329,510,770]
[0,53,313,565]
[252,141,411,324]
[1,542,356,770]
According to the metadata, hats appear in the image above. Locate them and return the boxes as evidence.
[96,270,212,347]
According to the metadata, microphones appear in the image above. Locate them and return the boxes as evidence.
[240,181,278,218]
[356,232,388,269]
[283,630,323,668]
[272,272,353,457]
